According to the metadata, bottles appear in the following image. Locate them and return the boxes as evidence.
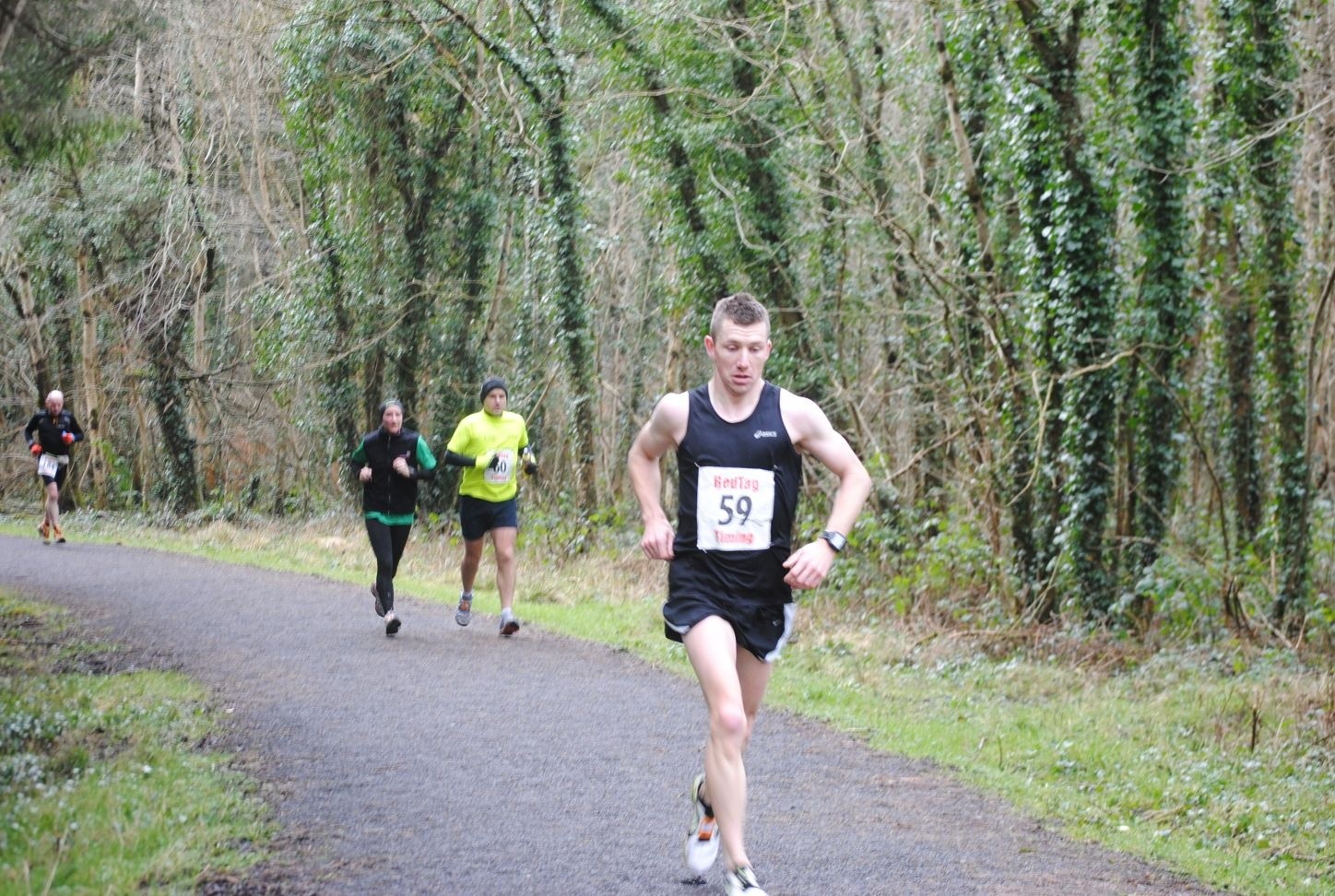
[522,447,531,479]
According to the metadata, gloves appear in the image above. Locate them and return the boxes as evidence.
[525,462,538,474]
[31,444,41,455]
[476,455,499,469]
[64,434,72,444]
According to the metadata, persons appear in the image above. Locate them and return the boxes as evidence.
[443,381,539,638]
[626,293,872,896]
[24,390,83,545]
[349,400,438,638]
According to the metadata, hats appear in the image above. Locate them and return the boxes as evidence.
[481,378,509,405]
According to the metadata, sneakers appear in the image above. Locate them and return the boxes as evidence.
[456,595,474,625]
[54,531,66,543]
[37,524,51,543]
[371,582,384,618]
[722,868,769,896]
[384,610,401,634]
[499,616,519,635]
[682,774,721,876]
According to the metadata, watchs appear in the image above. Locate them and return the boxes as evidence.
[818,530,848,552]
